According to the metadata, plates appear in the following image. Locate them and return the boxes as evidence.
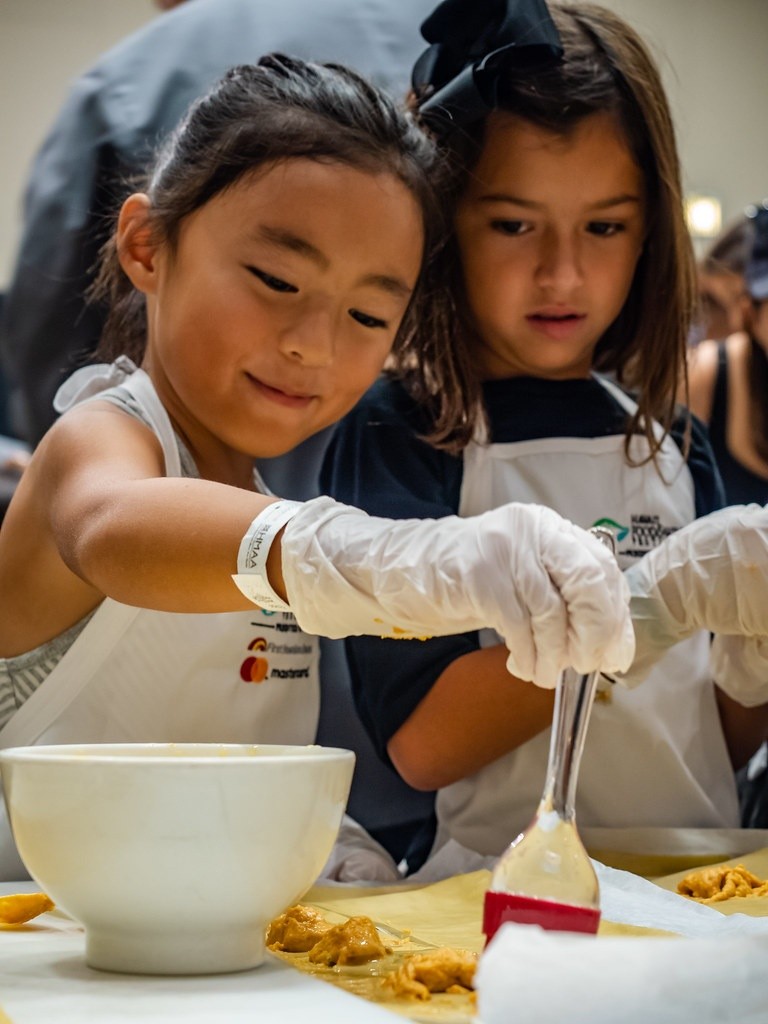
[574,827,768,880]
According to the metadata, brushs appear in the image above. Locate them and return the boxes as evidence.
[480,665,607,953]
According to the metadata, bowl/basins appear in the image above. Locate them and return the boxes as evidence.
[0,743,357,975]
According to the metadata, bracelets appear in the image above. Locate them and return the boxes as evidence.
[231,501,307,613]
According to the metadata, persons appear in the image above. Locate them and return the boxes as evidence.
[331,0,768,866]
[0,0,639,874]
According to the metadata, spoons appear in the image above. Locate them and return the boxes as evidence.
[481,525,616,952]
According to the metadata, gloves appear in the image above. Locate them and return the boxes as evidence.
[280,495,637,690]
[707,634,768,709]
[602,502,768,692]
